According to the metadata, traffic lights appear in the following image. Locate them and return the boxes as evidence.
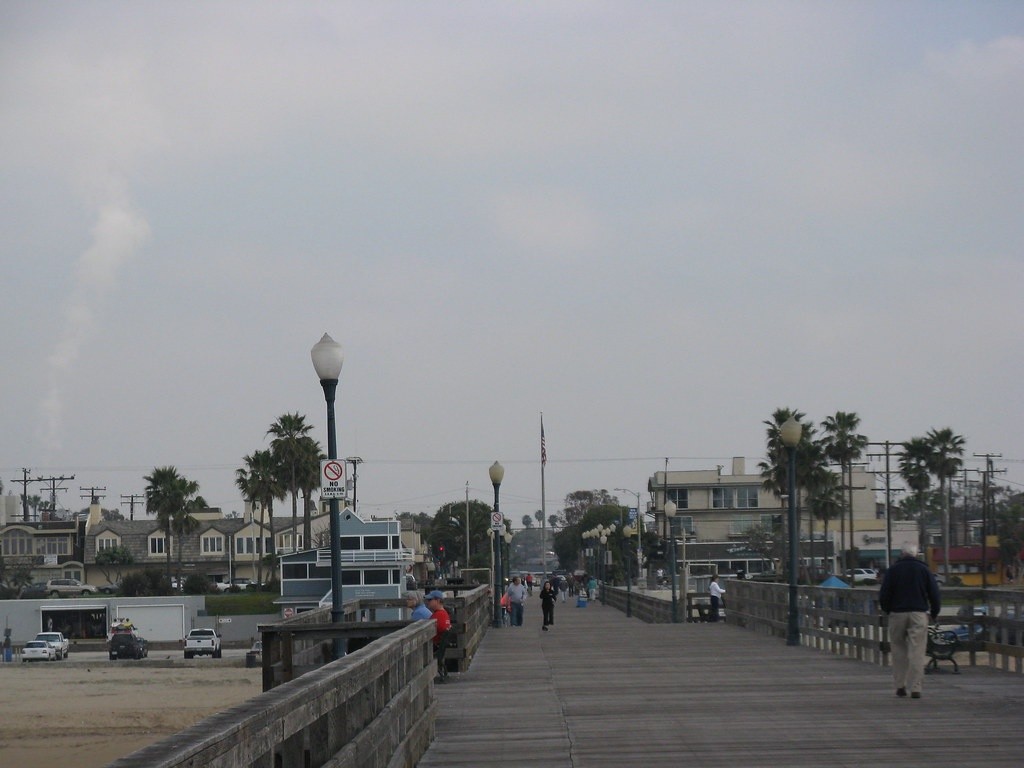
[440,545,443,555]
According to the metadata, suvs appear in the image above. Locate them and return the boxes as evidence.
[109,633,148,660]
[109,622,137,639]
[47,578,98,597]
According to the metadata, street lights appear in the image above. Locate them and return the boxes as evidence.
[614,488,642,575]
[488,460,512,628]
[310,332,349,659]
[581,523,632,617]
[780,416,802,644]
[665,500,679,623]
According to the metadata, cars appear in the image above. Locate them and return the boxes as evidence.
[251,640,262,663]
[21,641,56,661]
[172,579,185,590]
[844,568,876,584]
[23,582,47,592]
[217,577,265,594]
[940,605,1017,646]
[98,579,125,594]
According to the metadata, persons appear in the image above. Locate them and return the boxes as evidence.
[708,575,727,623]
[423,590,452,652]
[879,543,942,698]
[406,591,433,620]
[47,614,53,632]
[503,573,598,630]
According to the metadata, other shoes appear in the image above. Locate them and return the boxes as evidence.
[897,687,905,696]
[911,692,921,698]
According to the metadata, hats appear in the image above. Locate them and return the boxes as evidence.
[561,577,566,580]
[423,589,442,599]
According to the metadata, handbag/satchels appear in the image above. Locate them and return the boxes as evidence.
[552,598,556,608]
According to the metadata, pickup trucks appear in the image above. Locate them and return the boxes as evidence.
[184,629,222,658]
[34,632,69,659]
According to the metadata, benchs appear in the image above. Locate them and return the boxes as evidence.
[434,627,454,681]
[926,627,963,676]
